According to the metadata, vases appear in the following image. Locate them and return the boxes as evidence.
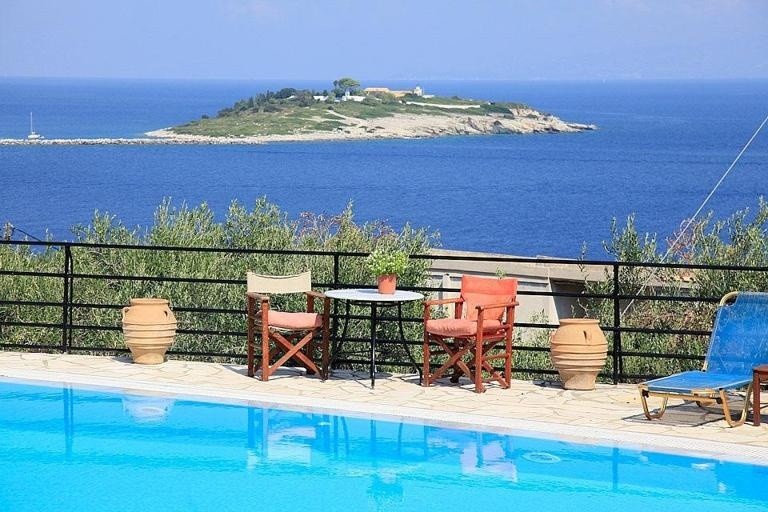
[120,296,178,366]
[549,317,608,390]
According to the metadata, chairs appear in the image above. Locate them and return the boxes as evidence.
[421,273,519,393]
[245,270,331,381]
[638,290,768,427]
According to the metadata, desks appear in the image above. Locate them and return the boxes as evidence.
[324,288,425,390]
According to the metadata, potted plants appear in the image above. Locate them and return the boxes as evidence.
[361,240,409,296]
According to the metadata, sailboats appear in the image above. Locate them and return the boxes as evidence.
[28,112,44,139]
